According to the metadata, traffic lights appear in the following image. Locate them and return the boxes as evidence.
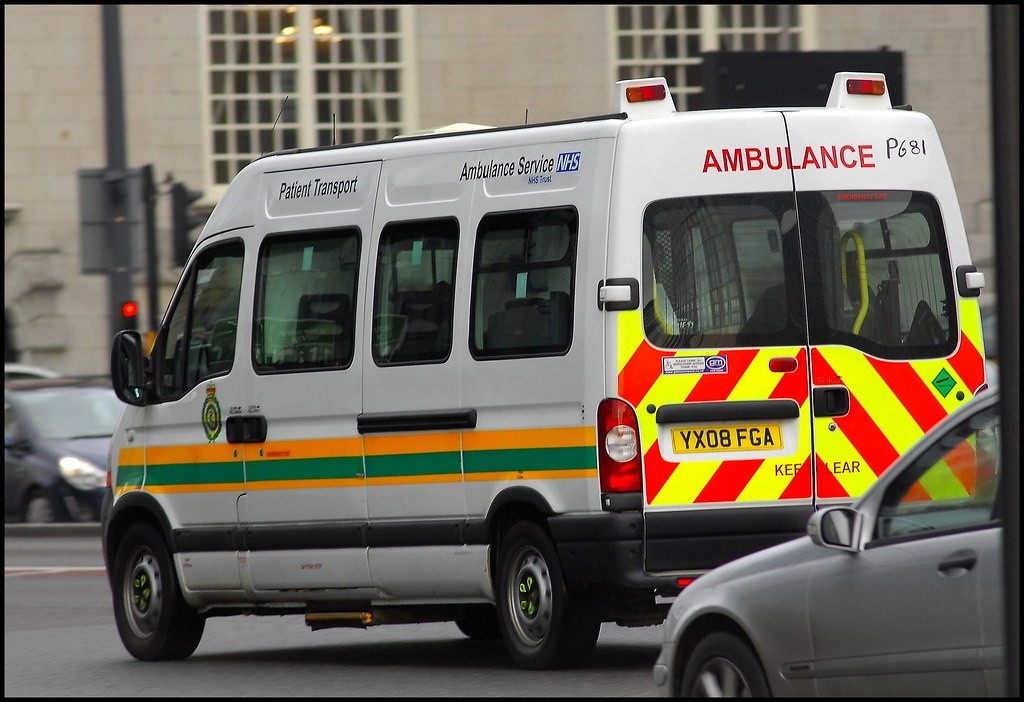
[122,300,139,330]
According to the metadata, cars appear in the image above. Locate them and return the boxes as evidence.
[651,388,1020,698]
[4,361,134,525]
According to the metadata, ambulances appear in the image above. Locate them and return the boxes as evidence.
[102,71,987,661]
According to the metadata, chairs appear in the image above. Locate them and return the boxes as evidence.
[283,291,450,363]
